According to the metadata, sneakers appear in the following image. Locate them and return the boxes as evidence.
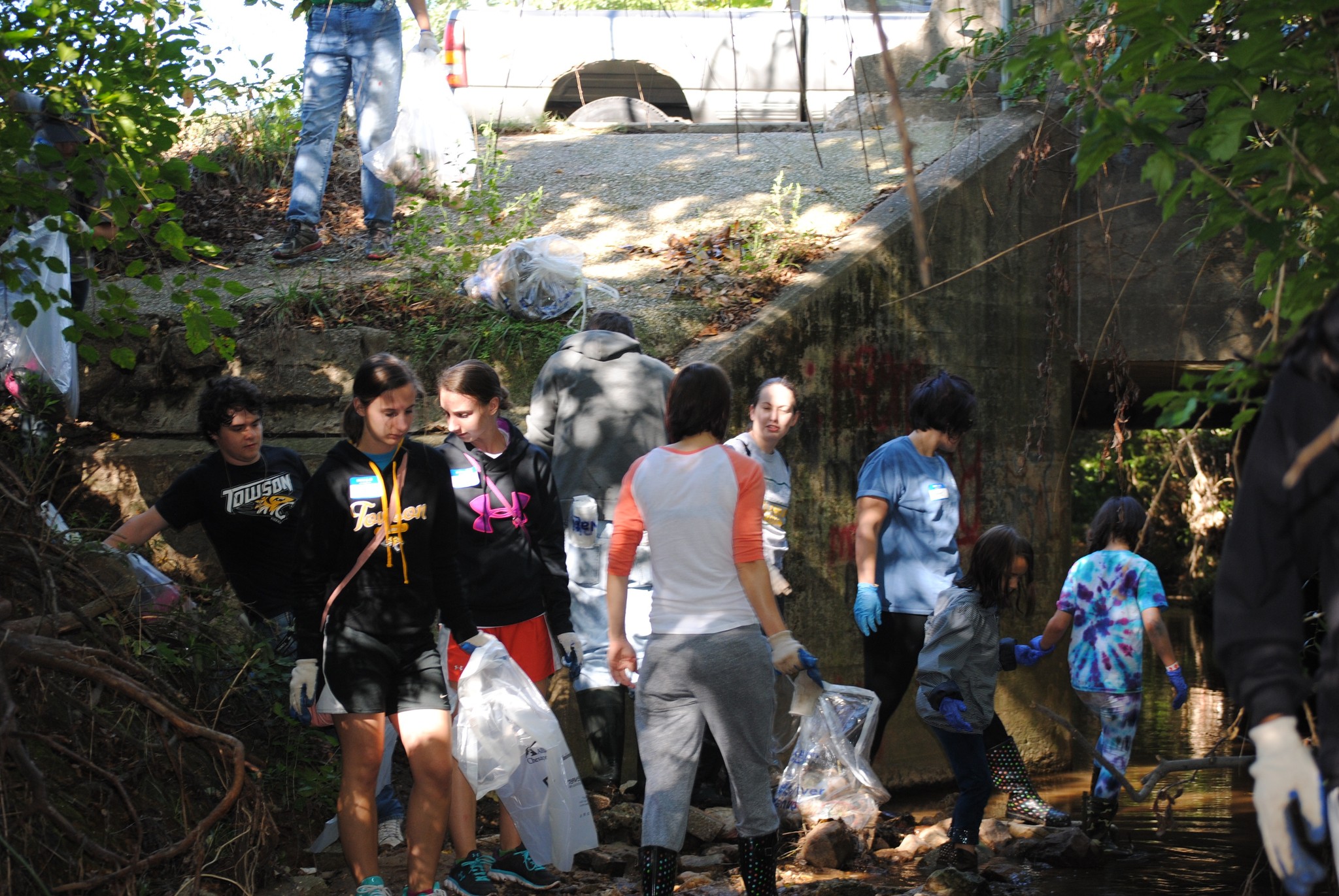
[402,882,446,896]
[364,223,395,260]
[487,844,561,891]
[355,875,392,896]
[443,849,498,896]
[271,222,322,260]
[378,819,404,848]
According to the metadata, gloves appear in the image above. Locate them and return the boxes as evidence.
[1166,667,1188,711]
[1014,645,1042,667]
[852,582,883,636]
[1028,635,1054,655]
[937,696,973,733]
[766,630,810,677]
[288,658,317,714]
[417,29,441,54]
[1248,717,1327,896]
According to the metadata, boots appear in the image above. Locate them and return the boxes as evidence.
[737,830,781,896]
[1080,791,1134,859]
[638,845,677,896]
[984,736,1072,827]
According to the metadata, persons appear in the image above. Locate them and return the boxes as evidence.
[15,125,118,317]
[269,0,442,258]
[93,311,1193,895]
[1213,289,1339,896]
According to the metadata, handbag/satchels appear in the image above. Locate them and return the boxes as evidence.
[457,234,620,334]
[308,701,334,727]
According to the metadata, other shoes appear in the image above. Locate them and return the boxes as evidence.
[935,842,978,873]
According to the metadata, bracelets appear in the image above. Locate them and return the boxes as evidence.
[1166,661,1180,672]
[88,229,93,234]
